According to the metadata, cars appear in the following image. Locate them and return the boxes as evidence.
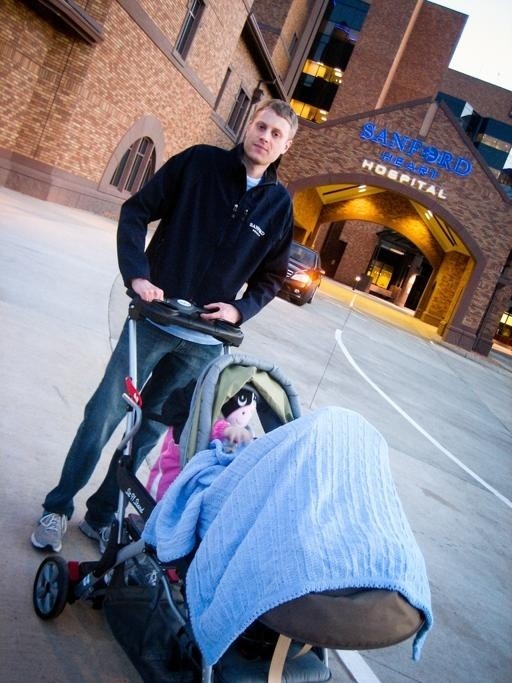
[277,237,330,308]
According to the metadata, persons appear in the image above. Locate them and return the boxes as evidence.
[29,97,302,554]
[200,417,262,485]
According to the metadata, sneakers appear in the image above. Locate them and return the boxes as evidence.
[78,515,113,555]
[29,509,68,554]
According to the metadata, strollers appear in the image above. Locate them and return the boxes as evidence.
[27,292,434,681]
